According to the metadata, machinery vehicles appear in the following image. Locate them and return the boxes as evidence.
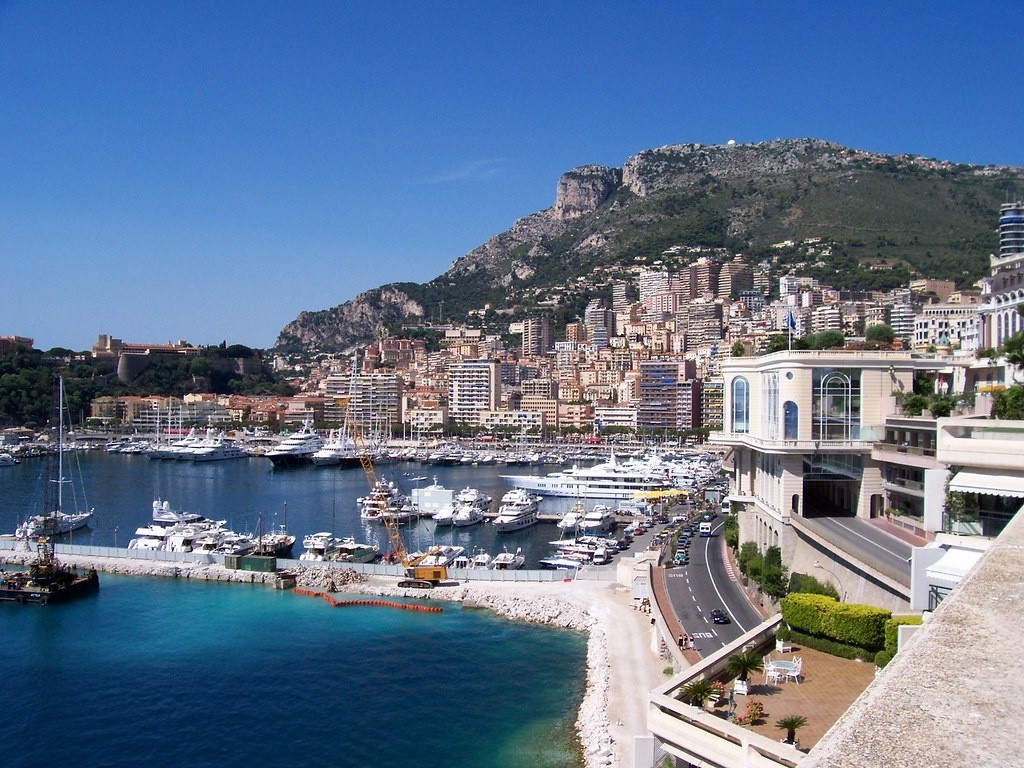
[336,397,446,588]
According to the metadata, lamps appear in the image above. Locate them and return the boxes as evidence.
[988,357,997,369]
[888,365,894,374]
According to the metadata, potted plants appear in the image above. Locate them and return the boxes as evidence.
[944,468,983,535]
[727,650,764,696]
[776,626,791,653]
[710,691,724,701]
[774,715,809,749]
[681,680,713,711]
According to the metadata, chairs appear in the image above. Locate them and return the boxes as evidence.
[762,654,802,686]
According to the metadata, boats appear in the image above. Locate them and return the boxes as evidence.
[127,498,248,563]
[105,403,248,463]
[250,415,724,570]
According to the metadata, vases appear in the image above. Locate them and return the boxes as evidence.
[713,689,723,691]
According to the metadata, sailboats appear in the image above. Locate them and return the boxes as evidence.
[15,377,94,538]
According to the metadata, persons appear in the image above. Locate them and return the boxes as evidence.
[677,634,683,651]
[689,635,695,650]
[683,633,687,650]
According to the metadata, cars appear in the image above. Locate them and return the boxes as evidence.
[593,476,729,565]
[710,608,731,624]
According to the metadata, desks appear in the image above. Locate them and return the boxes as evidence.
[771,661,795,680]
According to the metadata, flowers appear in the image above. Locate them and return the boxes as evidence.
[711,680,723,689]
[733,698,764,726]
